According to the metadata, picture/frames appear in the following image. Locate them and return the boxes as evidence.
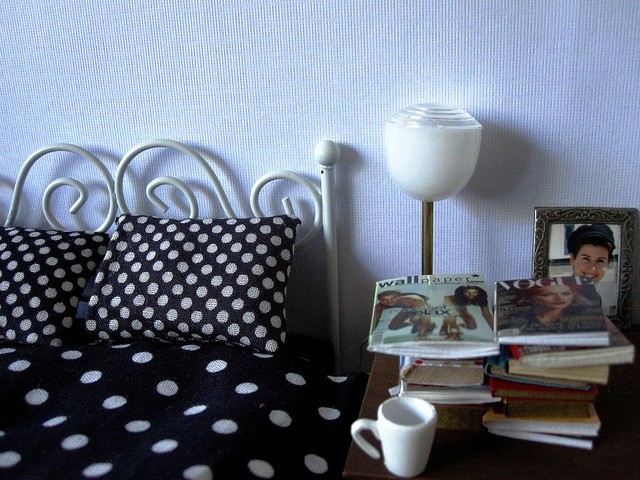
[532,206,638,333]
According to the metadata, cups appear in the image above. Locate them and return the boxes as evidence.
[350,397,438,478]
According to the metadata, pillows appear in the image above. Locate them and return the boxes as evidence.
[0,225,110,353]
[83,214,302,358]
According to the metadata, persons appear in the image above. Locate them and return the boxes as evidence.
[371,290,436,339]
[504,280,603,331]
[566,223,616,286]
[440,282,493,341]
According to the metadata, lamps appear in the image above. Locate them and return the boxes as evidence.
[381,102,484,274]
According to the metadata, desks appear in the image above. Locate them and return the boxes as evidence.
[340,352,640,480]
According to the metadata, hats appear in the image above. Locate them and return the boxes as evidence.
[568,222,615,256]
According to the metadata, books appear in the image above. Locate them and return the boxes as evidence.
[365,273,635,453]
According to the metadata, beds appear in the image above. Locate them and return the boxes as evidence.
[0,139,354,480]
